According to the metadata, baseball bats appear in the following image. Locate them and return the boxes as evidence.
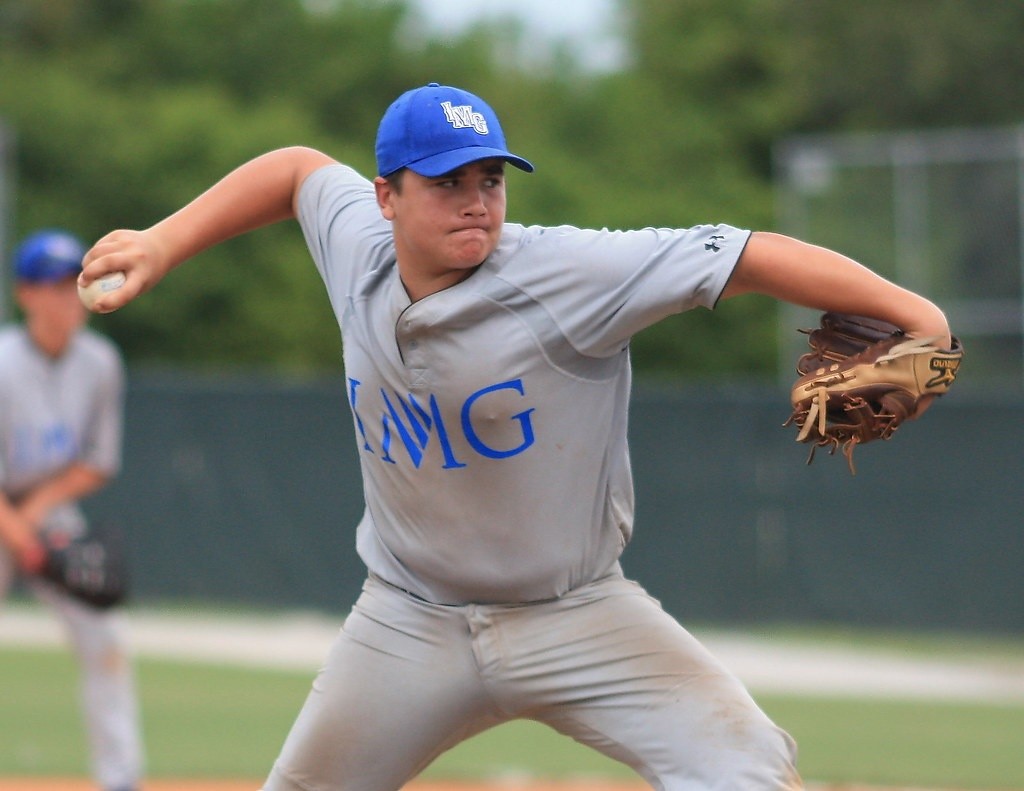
[792,306,966,455]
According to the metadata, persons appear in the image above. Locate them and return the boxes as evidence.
[2,231,142,790]
[76,84,964,790]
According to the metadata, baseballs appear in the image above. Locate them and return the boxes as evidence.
[76,269,126,314]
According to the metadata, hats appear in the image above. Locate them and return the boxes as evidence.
[14,232,88,281]
[375,83,534,177]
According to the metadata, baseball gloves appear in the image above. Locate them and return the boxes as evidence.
[32,504,130,615]
